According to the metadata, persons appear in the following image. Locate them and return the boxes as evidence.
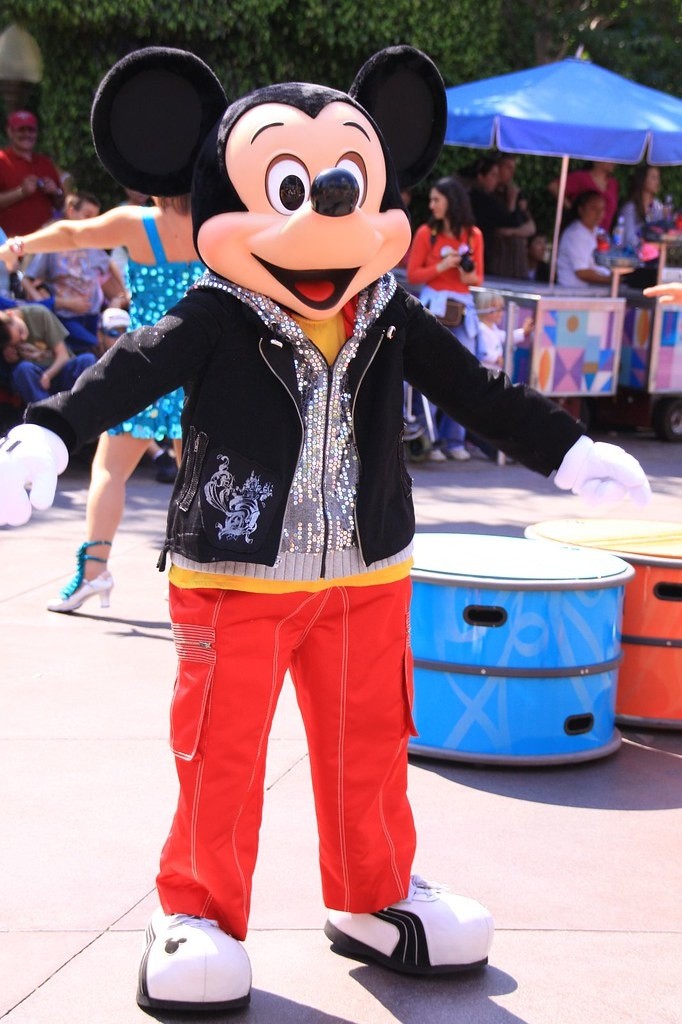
[0,109,682,611]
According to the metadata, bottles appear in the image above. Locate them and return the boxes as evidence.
[613,216,626,245]
[662,194,673,219]
[596,227,610,254]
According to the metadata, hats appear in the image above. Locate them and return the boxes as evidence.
[103,308,132,328]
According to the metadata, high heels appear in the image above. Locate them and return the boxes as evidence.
[47,540,113,611]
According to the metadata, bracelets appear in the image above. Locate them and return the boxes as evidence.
[6,237,24,257]
[51,188,64,200]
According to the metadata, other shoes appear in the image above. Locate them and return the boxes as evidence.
[148,452,178,481]
[429,450,446,461]
[447,445,470,460]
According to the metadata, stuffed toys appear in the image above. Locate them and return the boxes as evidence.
[0,46,652,1012]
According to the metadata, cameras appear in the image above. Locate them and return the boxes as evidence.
[460,250,474,273]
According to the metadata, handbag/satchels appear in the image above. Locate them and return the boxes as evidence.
[426,301,462,326]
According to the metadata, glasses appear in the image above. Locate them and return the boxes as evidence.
[101,329,121,338]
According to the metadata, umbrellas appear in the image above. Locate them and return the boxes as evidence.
[444,57,682,291]
